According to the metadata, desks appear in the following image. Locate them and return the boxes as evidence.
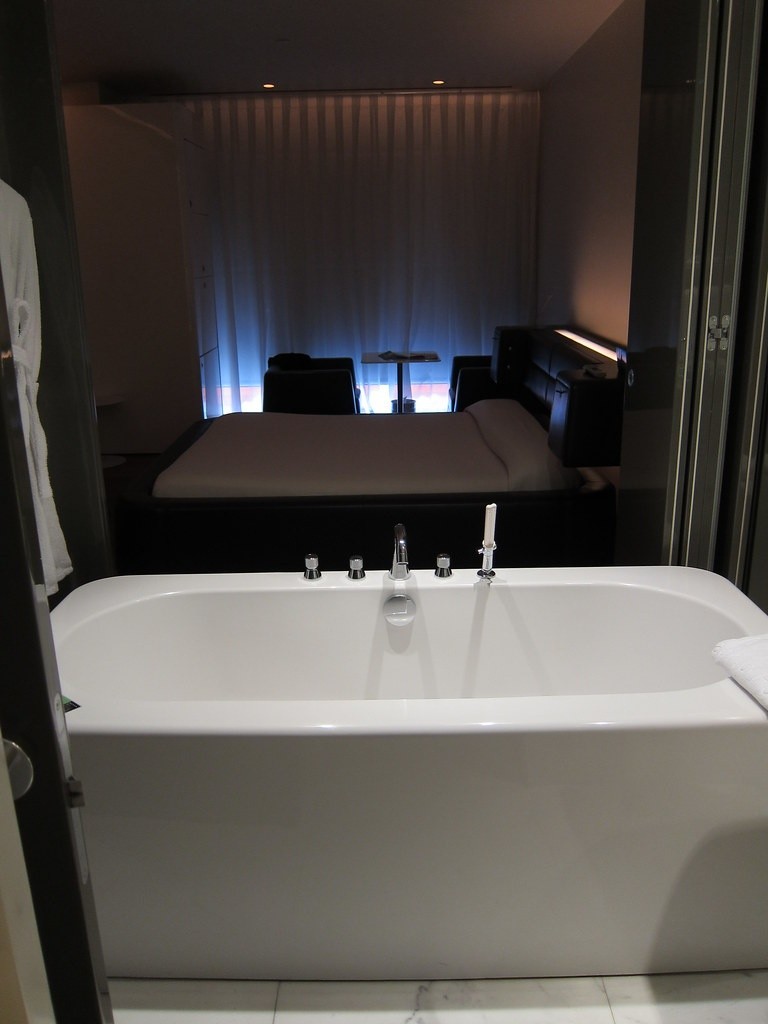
[360,350,441,414]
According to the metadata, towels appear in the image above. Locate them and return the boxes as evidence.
[712,632,768,712]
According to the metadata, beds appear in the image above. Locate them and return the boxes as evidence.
[120,324,630,572]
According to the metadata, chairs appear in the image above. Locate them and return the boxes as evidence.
[450,355,495,412]
[263,352,361,415]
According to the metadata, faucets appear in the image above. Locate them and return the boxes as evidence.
[387,522,411,580]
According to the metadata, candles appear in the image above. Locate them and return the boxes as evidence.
[483,501,497,541]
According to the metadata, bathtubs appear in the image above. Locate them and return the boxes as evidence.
[47,565,768,983]
